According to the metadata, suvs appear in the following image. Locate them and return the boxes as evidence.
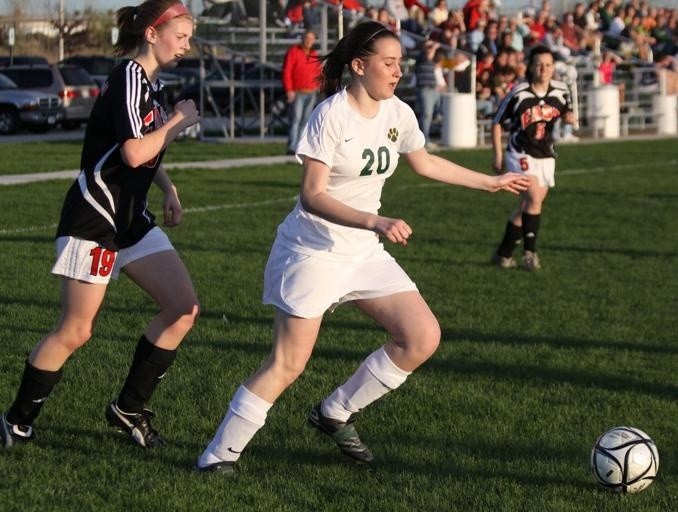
[56,55,130,96]
[0,73,65,134]
[166,58,228,105]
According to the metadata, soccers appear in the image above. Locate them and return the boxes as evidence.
[591,425,660,493]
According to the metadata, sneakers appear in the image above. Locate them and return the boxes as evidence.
[521,250,540,270]
[307,402,374,463]
[198,456,240,481]
[492,252,516,268]
[105,400,166,447]
[0,411,35,449]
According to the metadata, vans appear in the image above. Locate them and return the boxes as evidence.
[0,64,101,127]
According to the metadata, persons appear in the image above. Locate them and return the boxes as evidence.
[0,1,201,449]
[268,1,678,113]
[282,32,321,156]
[489,43,578,271]
[196,19,532,478]
